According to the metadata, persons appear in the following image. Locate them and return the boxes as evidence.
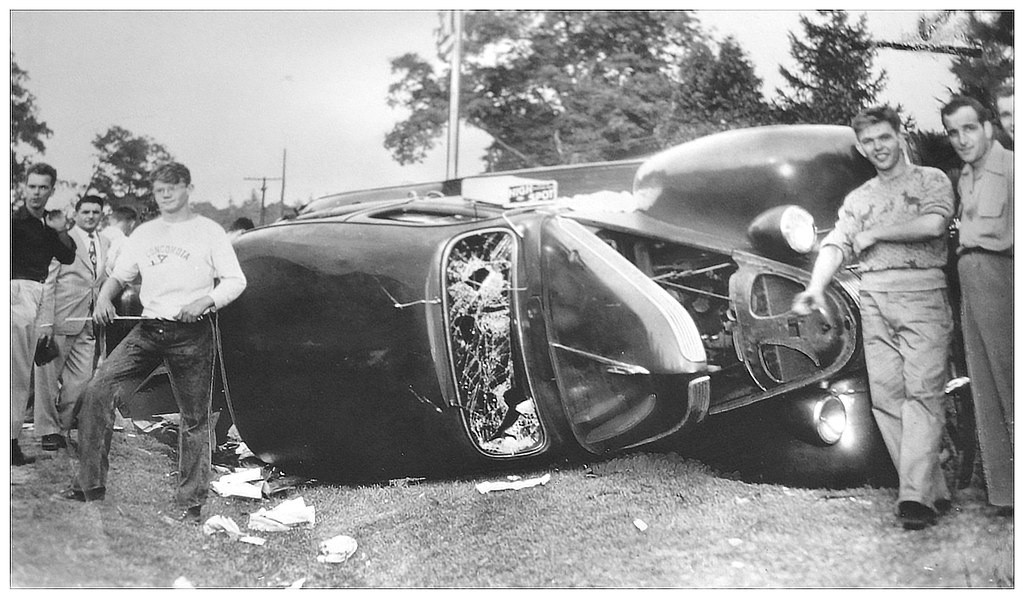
[62,162,247,526]
[10,162,255,465]
[790,94,1015,527]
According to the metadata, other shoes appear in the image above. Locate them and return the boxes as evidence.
[42,433,77,451]
[161,505,201,528]
[12,439,36,466]
[899,499,951,529]
[50,485,106,503]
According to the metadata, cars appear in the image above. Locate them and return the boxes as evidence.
[216,121,916,493]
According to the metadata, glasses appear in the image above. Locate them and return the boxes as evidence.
[152,185,185,194]
